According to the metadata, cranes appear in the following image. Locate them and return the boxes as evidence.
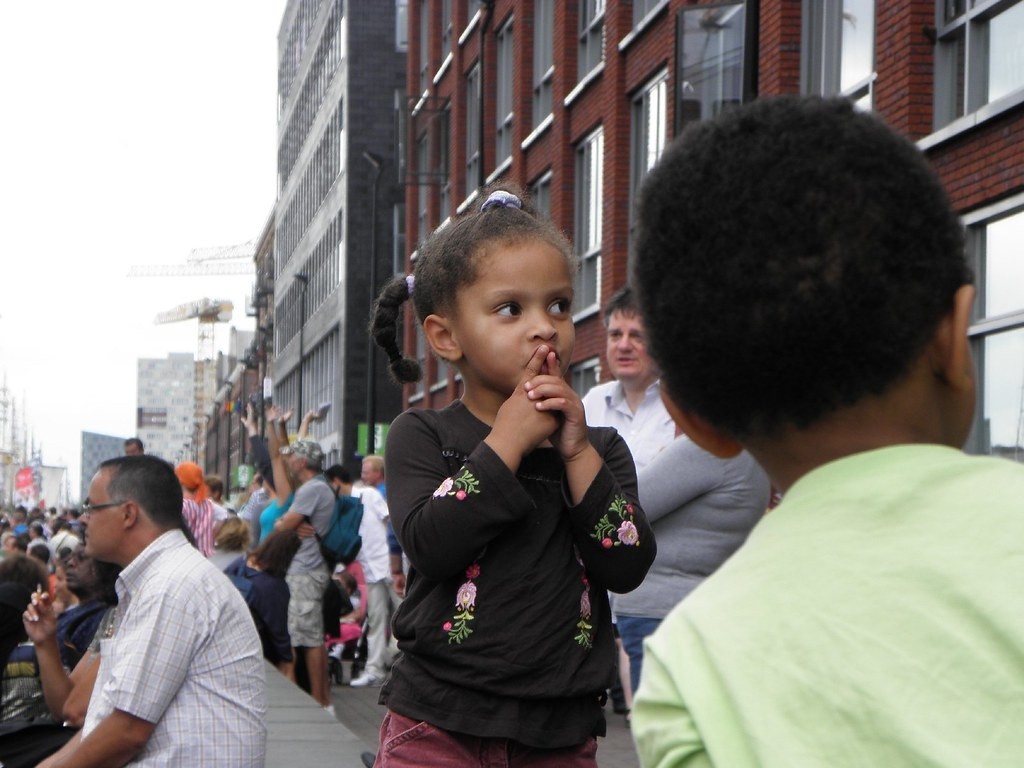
[156,296,234,468]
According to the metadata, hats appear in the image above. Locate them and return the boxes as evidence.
[278,439,325,462]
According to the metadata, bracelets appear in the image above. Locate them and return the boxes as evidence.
[391,570,403,574]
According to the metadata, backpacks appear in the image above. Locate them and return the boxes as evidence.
[302,475,364,568]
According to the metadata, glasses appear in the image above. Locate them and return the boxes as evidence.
[78,497,128,517]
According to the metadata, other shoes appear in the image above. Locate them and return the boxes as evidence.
[349,670,384,687]
[360,752,376,768]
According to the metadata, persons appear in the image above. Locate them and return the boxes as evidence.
[24,454,267,768]
[326,466,393,687]
[362,455,388,500]
[369,189,652,768]
[582,287,676,476]
[385,515,407,600]
[612,430,768,697]
[630,96,1024,768]
[249,437,339,713]
[0,403,320,720]
